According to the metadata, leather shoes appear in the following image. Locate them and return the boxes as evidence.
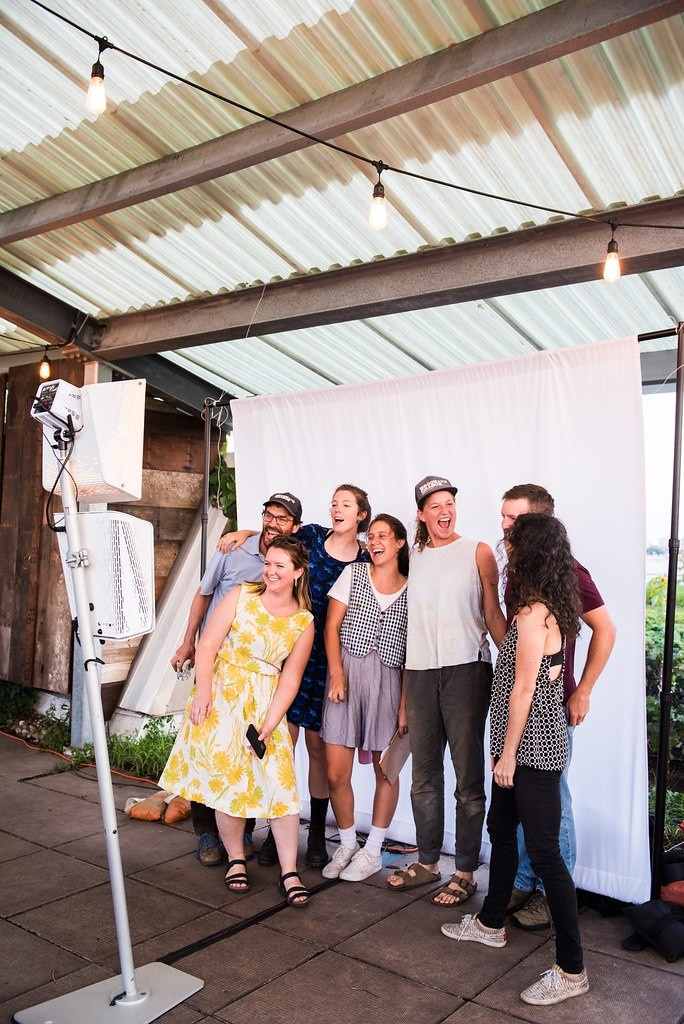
[197,833,222,867]
[243,832,255,861]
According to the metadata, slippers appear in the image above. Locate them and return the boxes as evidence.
[431,874,477,907]
[388,863,442,891]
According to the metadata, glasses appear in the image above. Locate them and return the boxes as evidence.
[262,510,294,526]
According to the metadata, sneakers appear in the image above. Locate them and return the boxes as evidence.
[520,964,589,1005]
[322,842,360,879]
[507,886,536,913]
[441,912,507,947]
[339,848,382,881]
[514,894,553,929]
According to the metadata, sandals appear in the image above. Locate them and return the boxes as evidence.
[224,859,251,893]
[278,873,309,908]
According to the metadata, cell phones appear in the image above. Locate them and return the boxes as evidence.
[246,724,266,760]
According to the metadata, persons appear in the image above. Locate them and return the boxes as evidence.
[319,512,409,883]
[173,491,303,867]
[499,482,616,929]
[385,476,510,907]
[217,483,371,869]
[170,536,314,907]
[440,512,588,1005]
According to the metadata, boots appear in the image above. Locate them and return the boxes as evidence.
[258,824,279,867]
[305,823,328,868]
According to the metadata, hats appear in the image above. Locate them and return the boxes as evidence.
[415,476,458,504]
[263,492,302,519]
